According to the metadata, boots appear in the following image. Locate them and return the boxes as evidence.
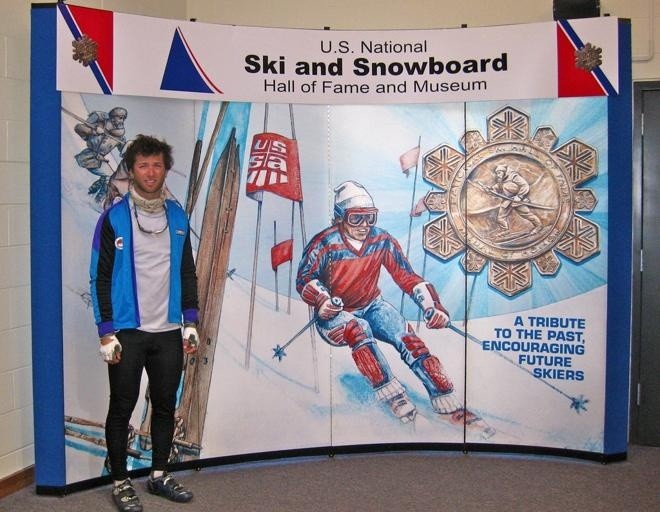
[438,407,486,433]
[386,390,415,418]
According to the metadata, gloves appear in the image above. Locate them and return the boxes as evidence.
[411,281,451,329]
[181,326,200,353]
[97,334,122,362]
[300,278,343,321]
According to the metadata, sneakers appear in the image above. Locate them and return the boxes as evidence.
[111,479,144,512]
[146,471,194,502]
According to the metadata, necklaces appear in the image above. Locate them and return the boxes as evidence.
[133,203,169,235]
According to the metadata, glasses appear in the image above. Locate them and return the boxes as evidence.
[134,210,171,236]
[342,208,378,227]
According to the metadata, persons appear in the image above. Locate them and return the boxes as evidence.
[292,180,478,426]
[89,135,201,511]
[73,107,129,209]
